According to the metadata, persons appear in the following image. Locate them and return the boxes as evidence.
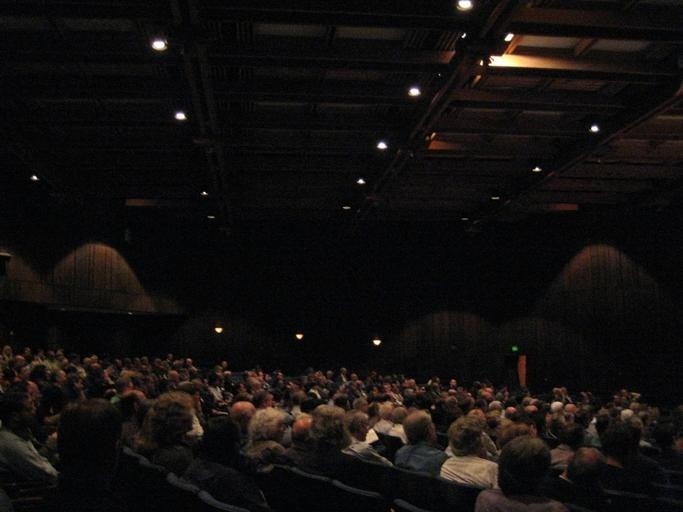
[1,344,683,512]
